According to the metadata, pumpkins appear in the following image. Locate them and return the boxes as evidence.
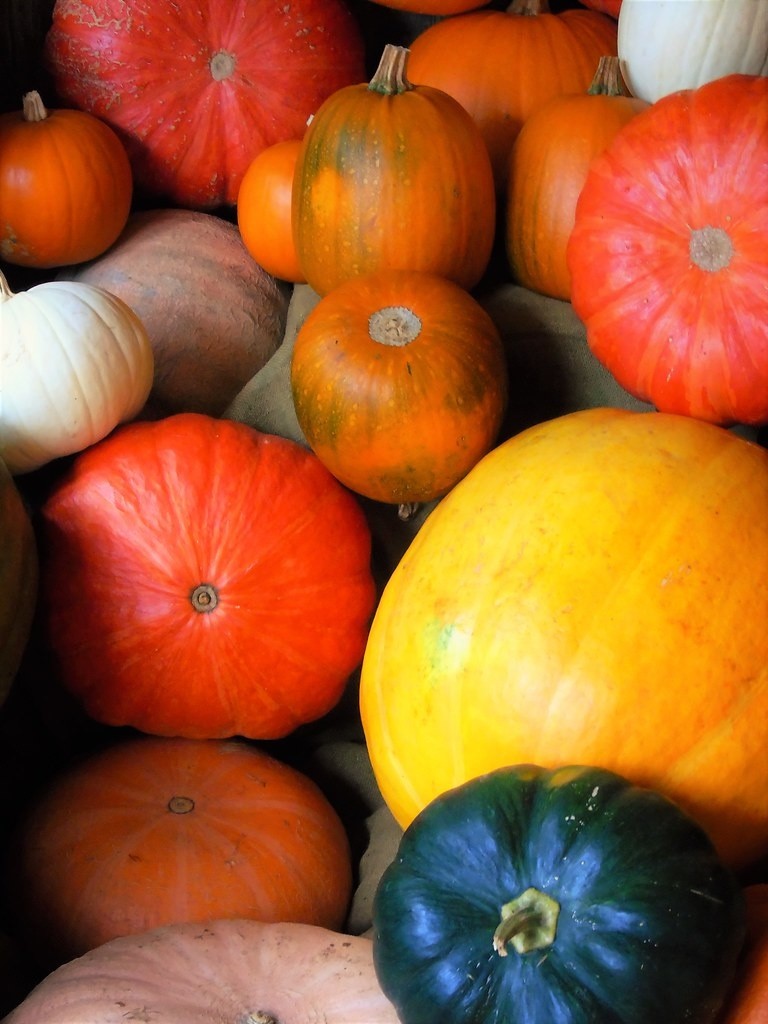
[0,0,767,1022]
